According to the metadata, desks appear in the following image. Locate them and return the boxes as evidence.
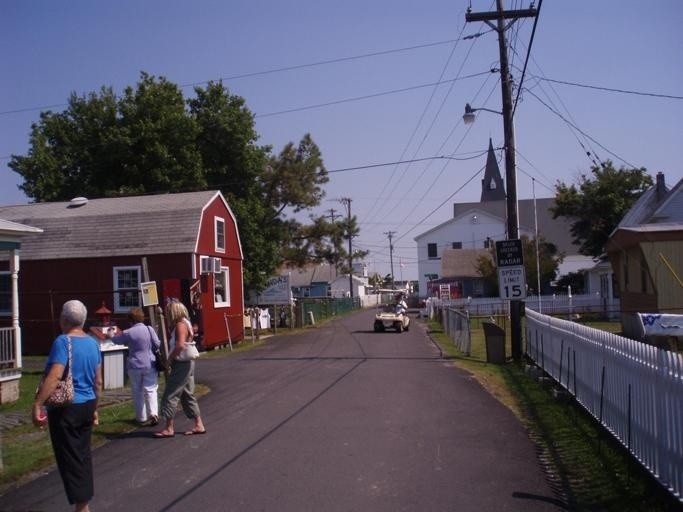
[461,102,523,364]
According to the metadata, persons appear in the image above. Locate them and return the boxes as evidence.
[392,294,408,315]
[112,306,159,425]
[32,299,104,511]
[152,299,205,439]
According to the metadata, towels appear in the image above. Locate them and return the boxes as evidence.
[482,321,505,364]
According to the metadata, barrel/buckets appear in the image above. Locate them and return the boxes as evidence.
[151,429,176,438]
[184,427,206,435]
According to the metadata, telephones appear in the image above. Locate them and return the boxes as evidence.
[498,266,527,300]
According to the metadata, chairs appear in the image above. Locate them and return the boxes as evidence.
[150,415,160,426]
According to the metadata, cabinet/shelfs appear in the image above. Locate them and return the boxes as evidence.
[38,406,48,433]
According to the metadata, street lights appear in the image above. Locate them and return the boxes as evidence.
[35,335,75,405]
[173,321,200,362]
[147,327,170,372]
